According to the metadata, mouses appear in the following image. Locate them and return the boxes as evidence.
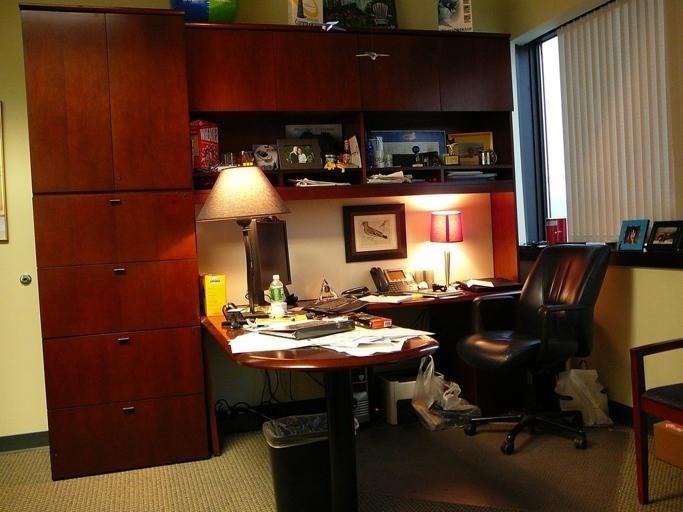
[344,294,357,300]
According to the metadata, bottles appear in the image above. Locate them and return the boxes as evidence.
[270,274,285,319]
[375,150,394,168]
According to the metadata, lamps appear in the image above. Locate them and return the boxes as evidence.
[431,210,464,291]
[196,165,292,319]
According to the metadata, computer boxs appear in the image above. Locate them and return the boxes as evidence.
[349,367,371,423]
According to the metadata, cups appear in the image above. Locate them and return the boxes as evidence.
[478,150,498,166]
[225,151,255,166]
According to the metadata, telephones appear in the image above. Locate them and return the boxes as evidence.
[369,267,410,294]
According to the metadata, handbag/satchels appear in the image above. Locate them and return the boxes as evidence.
[554,359,613,427]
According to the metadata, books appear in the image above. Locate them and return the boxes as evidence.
[357,292,412,305]
[400,289,463,299]
[364,170,413,185]
[285,177,353,187]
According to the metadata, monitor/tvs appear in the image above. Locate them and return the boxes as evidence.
[247,219,292,304]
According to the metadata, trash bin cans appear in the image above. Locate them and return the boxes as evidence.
[263,412,360,512]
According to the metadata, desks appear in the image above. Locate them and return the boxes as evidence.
[201,277,522,511]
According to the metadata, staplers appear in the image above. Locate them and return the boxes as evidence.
[341,286,370,298]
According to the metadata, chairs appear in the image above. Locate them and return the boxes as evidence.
[456,244,611,455]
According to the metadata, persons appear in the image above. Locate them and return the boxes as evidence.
[296,147,307,164]
[289,146,298,164]
[629,229,635,243]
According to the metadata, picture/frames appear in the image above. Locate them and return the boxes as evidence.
[616,219,683,253]
[344,204,407,263]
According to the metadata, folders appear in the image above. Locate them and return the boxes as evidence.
[258,320,355,340]
